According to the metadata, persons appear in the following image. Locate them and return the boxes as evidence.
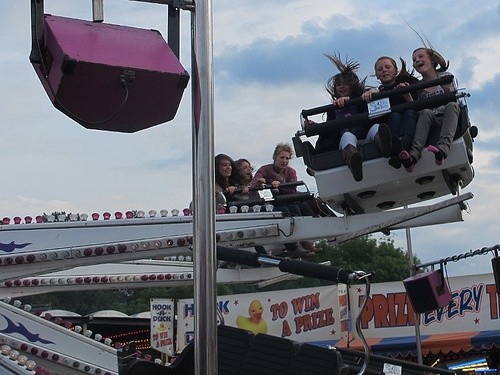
[214,143,324,267]
[360,18,468,173]
[304,51,393,182]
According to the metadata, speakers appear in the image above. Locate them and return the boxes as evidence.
[31,14,190,134]
[403,269,452,314]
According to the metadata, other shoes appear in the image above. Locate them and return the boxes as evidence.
[393,135,403,158]
[378,124,392,158]
[287,247,314,258]
[428,145,446,160]
[398,150,413,171]
[303,243,323,254]
[349,152,364,181]
